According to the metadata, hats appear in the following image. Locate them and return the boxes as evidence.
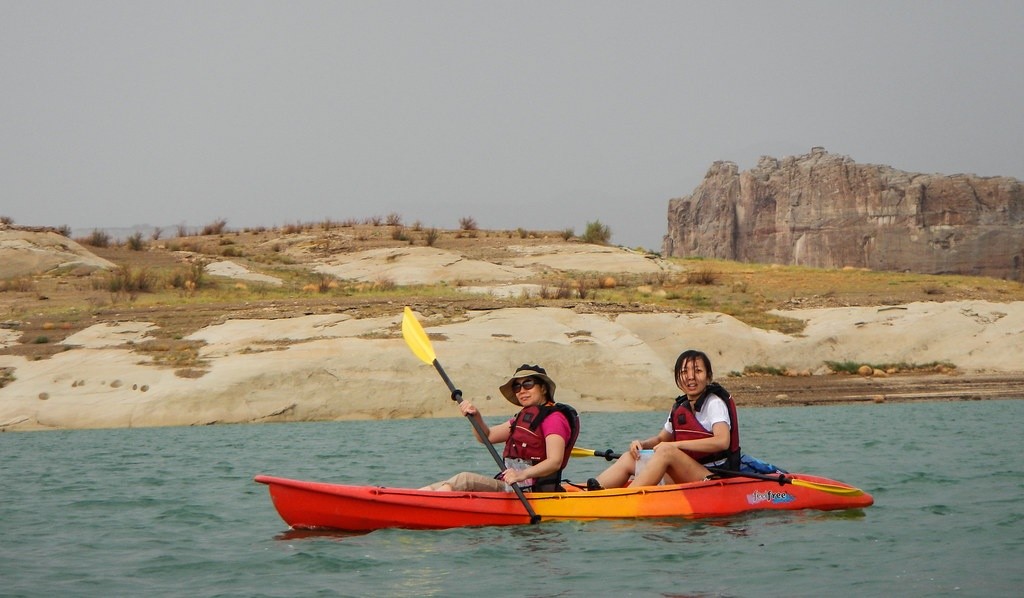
[498,362,555,407]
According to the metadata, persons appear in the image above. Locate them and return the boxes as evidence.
[587,350,732,490]
[421,364,572,492]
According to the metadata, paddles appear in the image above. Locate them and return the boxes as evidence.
[569,447,864,498]
[401,305,544,526]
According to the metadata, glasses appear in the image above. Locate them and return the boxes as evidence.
[511,380,542,392]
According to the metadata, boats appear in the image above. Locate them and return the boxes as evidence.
[252,471,877,534]
